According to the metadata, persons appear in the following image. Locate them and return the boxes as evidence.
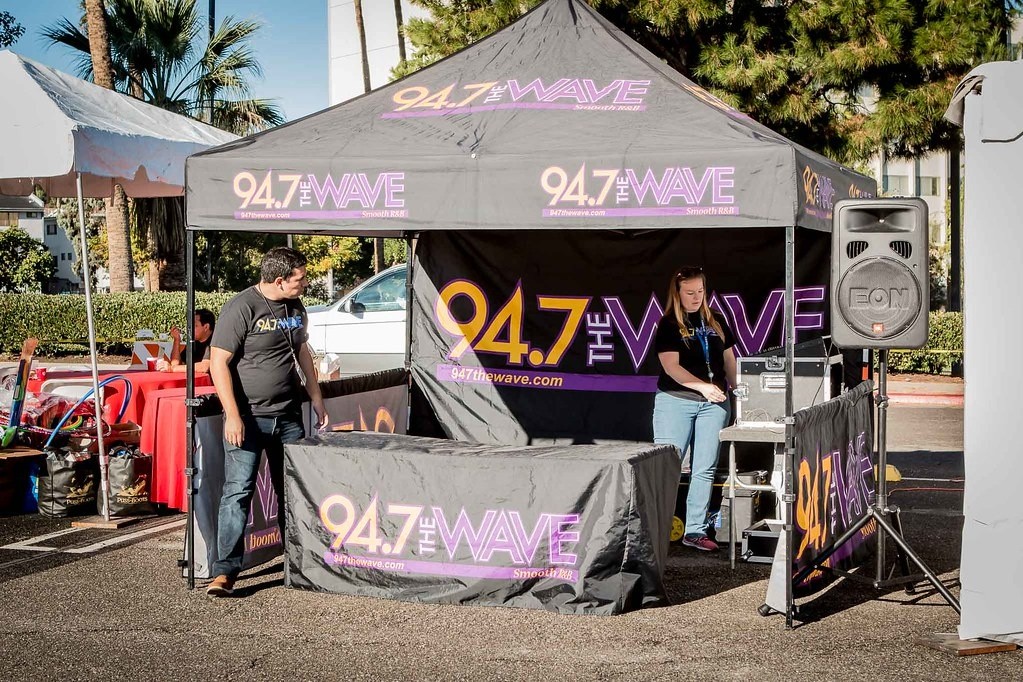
[207,247,329,594]
[156,308,216,386]
[652,265,737,551]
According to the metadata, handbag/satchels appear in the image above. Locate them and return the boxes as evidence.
[96,446,158,518]
[37,449,99,518]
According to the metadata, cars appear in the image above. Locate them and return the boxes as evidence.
[307,261,408,355]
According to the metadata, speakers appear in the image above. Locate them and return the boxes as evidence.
[831,196,930,348]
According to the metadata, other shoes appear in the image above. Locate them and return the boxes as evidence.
[207,575,234,594]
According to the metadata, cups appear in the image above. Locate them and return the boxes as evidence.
[36,367,46,381]
[146,358,158,372]
[159,334,167,342]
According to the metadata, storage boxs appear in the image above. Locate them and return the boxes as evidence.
[735,353,844,426]
[741,519,786,563]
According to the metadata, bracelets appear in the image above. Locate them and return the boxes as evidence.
[168,363,174,371]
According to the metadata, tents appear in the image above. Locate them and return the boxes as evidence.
[185,0,877,625]
[0,49,293,522]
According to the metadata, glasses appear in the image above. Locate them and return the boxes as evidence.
[676,266,703,280]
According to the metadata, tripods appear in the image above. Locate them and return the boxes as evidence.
[758,352,963,618]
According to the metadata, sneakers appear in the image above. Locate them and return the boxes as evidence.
[682,535,719,551]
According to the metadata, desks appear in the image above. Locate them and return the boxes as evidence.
[0,445,48,461]
[281,431,682,618]
[97,371,213,429]
[720,426,786,572]
[141,386,216,510]
